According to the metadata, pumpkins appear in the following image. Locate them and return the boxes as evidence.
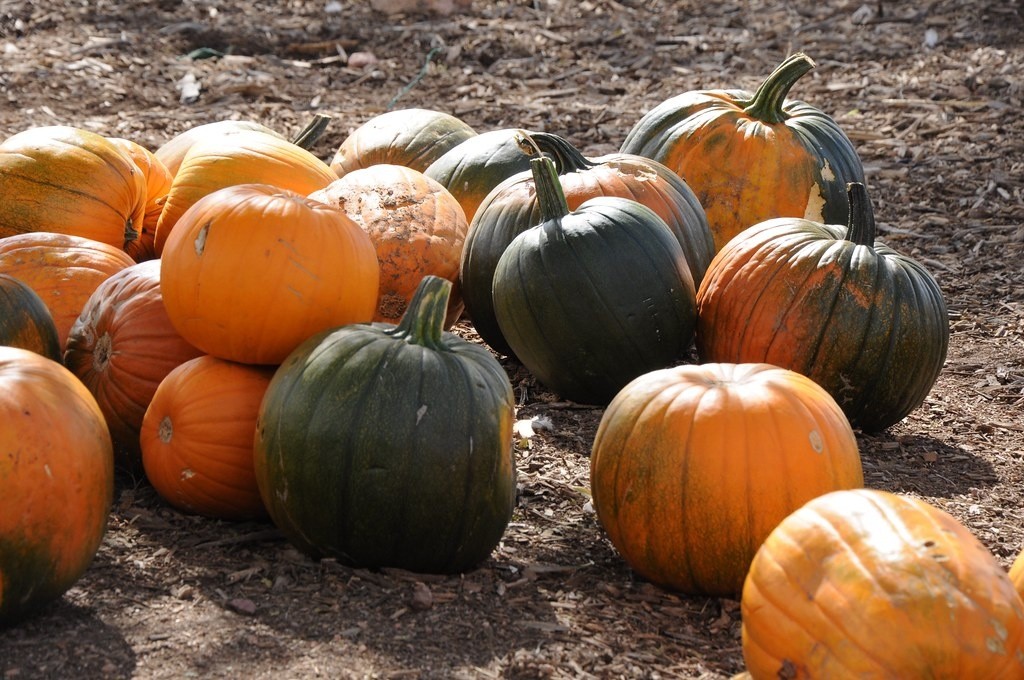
[2,54,1022,680]
